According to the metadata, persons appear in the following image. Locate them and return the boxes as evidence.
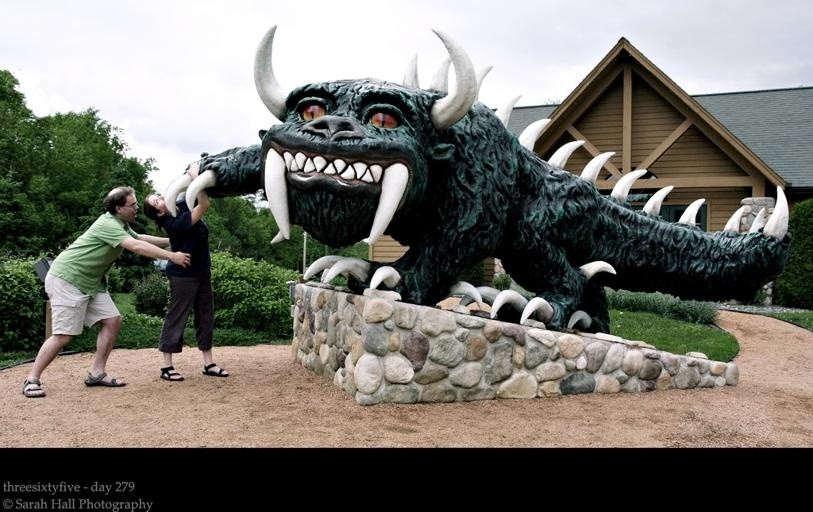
[21,183,192,399]
[141,158,229,382]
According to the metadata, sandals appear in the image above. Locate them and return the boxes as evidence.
[160,366,184,381]
[203,363,229,377]
[84,372,126,387]
[22,379,46,398]
